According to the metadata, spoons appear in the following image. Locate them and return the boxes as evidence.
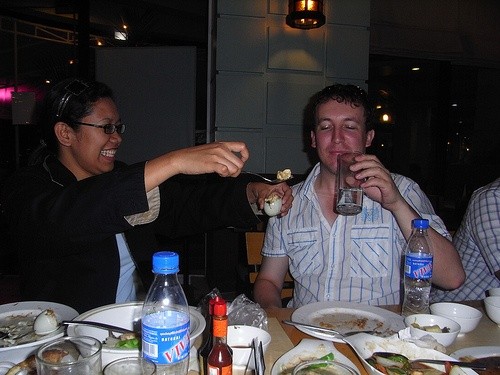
[241,171,295,185]
[372,352,487,372]
[282,320,382,337]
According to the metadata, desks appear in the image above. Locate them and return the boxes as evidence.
[49,300,500,375]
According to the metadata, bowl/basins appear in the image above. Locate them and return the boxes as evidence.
[66,301,206,369]
[483,288,500,329]
[430,302,483,338]
[404,314,461,348]
[227,325,271,370]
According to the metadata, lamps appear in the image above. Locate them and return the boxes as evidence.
[286,0,326,30]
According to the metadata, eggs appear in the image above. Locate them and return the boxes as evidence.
[265,197,282,216]
[34,310,60,334]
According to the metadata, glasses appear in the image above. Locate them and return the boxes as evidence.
[64,119,127,135]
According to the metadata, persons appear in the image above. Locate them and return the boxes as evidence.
[251,82,466,309]
[429,177,500,303]
[0,78,295,316]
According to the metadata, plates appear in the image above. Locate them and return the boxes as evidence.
[291,300,406,344]
[449,345,500,375]
[342,332,480,375]
[270,338,362,375]
[0,301,79,352]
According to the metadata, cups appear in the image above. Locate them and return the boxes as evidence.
[102,357,157,375]
[336,151,366,216]
[35,335,102,375]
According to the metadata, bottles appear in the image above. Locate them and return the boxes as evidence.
[198,295,233,375]
[401,219,433,318]
[140,251,191,375]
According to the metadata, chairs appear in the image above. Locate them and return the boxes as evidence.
[245,232,295,308]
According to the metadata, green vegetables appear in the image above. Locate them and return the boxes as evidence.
[115,338,142,348]
[309,353,333,369]
